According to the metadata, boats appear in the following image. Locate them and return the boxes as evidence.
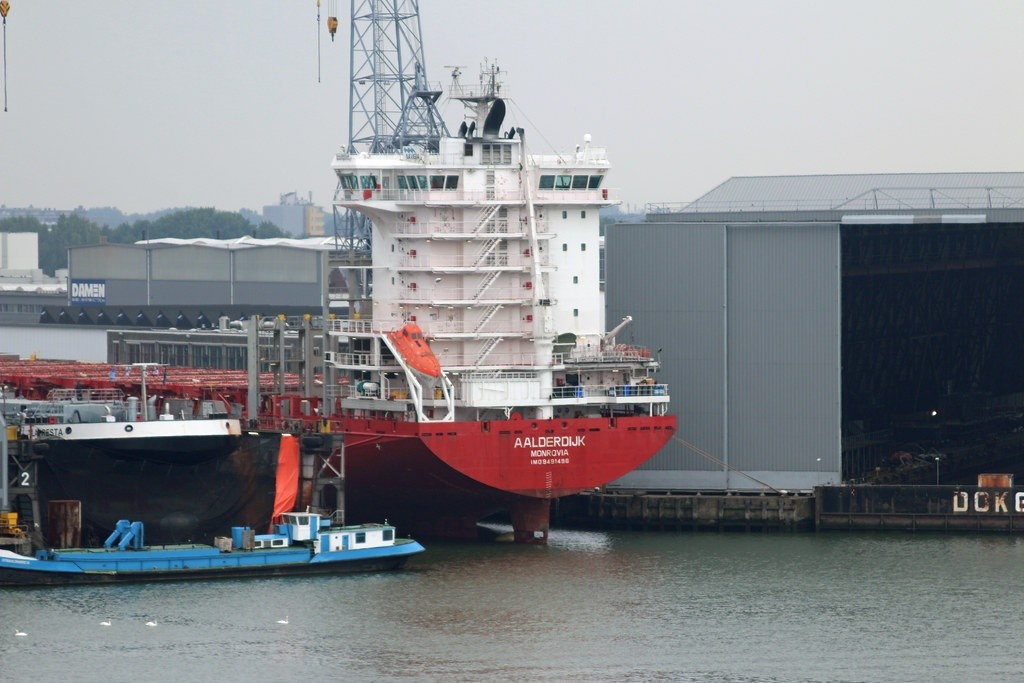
[0,58,678,546]
[1,511,426,586]
